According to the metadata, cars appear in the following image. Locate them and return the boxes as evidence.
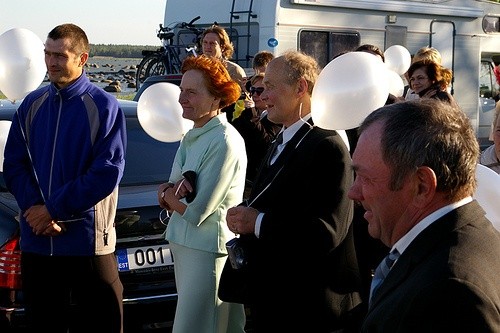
[0,97,185,329]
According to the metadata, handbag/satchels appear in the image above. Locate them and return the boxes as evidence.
[218,237,245,304]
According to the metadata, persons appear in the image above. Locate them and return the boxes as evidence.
[348,99,500,333]
[199,24,248,96]
[244,51,276,78]
[232,73,281,199]
[469,163,499,232]
[333,44,454,153]
[93,188,125,333]
[480,100,500,174]
[218,52,358,333]
[5,24,127,333]
[158,55,247,333]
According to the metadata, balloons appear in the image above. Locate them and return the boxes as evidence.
[310,51,391,130]
[0,121,12,172]
[0,27,47,103]
[137,82,195,142]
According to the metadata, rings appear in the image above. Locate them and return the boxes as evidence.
[235,225,237,231]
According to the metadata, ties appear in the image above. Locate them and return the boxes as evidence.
[369,248,399,311]
[263,131,285,167]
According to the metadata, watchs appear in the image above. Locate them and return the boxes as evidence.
[161,185,170,202]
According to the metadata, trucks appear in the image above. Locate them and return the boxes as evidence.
[163,0,500,150]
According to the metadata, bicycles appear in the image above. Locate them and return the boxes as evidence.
[135,16,223,92]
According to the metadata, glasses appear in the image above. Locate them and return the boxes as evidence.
[250,87,264,96]
[409,76,429,82]
